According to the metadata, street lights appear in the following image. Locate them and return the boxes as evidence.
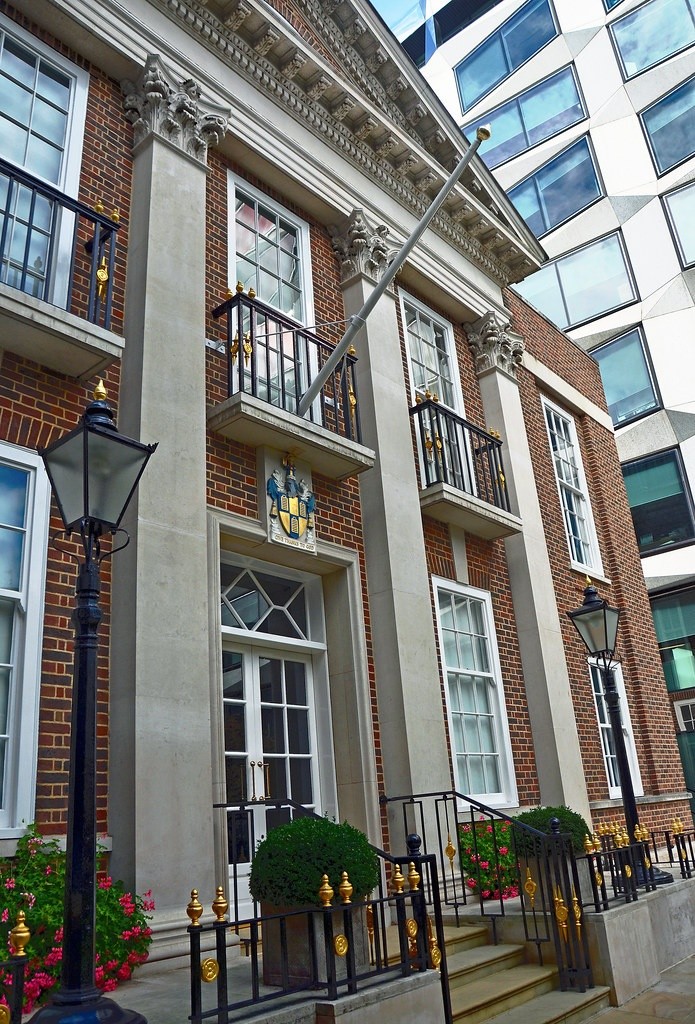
[26,378,159,1023]
[565,574,674,888]
